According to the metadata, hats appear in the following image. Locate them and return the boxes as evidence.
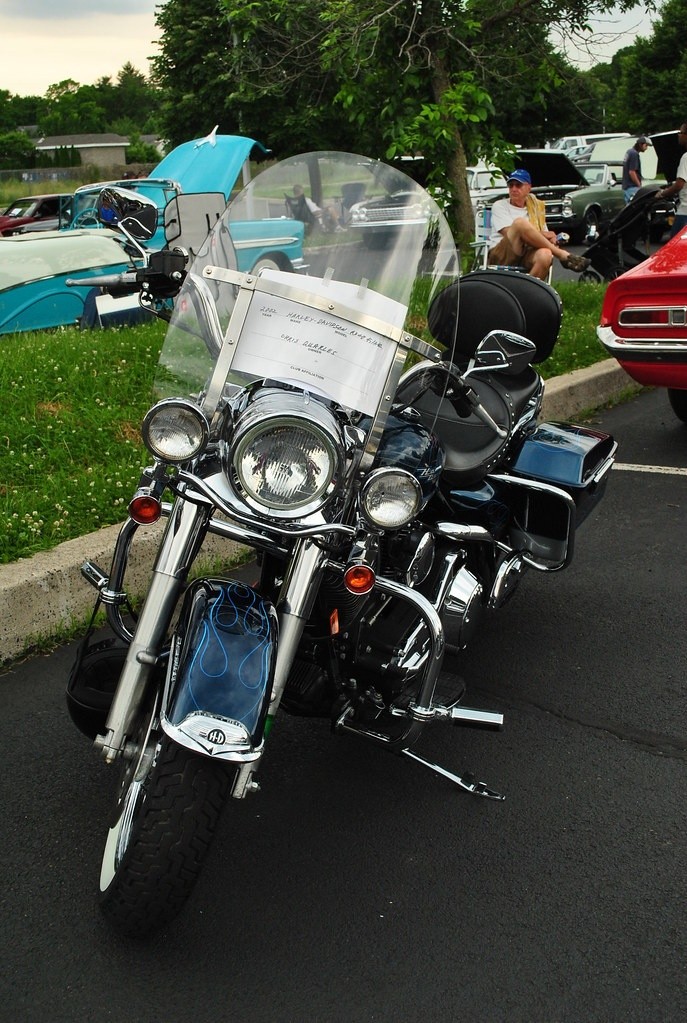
[506,169,531,184]
[293,185,305,198]
[638,137,653,146]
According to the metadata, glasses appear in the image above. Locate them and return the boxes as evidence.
[508,182,523,188]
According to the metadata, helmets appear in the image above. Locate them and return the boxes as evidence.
[66,647,156,742]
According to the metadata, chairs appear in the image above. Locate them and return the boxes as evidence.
[470,209,553,286]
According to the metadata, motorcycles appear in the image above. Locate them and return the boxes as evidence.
[64,147,618,941]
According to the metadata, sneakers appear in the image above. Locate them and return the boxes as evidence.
[560,253,591,272]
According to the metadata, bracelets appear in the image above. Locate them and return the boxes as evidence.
[660,190,666,198]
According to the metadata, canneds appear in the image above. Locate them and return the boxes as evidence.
[556,232,570,242]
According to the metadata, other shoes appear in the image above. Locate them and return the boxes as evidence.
[334,226,347,233]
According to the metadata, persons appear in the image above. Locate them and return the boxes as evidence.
[488,170,591,283]
[100,199,117,224]
[292,185,349,233]
[622,136,651,207]
[655,123,687,241]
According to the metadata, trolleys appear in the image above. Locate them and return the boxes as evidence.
[579,186,677,284]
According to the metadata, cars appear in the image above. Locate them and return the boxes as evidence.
[0,193,100,239]
[0,124,311,336]
[596,223,686,424]
[349,132,687,250]
